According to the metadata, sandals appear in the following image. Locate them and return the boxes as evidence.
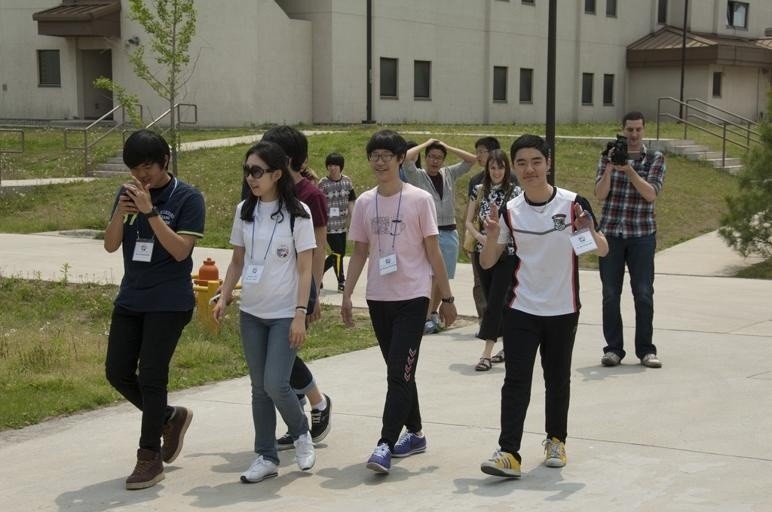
[491,350,506,363]
[475,357,492,371]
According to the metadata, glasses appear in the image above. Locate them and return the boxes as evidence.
[243,164,275,178]
[369,151,395,162]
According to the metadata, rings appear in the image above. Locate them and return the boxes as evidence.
[136,192,139,196]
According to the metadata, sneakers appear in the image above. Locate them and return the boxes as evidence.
[366,442,390,475]
[240,454,278,483]
[424,312,442,334]
[163,406,194,464]
[641,354,663,368]
[310,393,332,443]
[294,431,316,471]
[391,431,427,458]
[337,285,345,293]
[275,432,294,450]
[601,351,621,367]
[481,450,522,478]
[543,437,567,468]
[126,448,165,489]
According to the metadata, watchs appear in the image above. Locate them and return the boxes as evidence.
[143,206,159,219]
[442,297,454,303]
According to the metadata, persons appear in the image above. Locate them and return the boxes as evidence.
[478,132,611,479]
[461,149,523,371]
[468,137,517,339]
[302,154,325,290]
[103,128,206,491]
[211,140,319,486]
[263,125,332,453]
[593,109,665,369]
[340,129,458,475]
[401,138,478,334]
[314,153,356,295]
[397,142,421,182]
[301,136,523,373]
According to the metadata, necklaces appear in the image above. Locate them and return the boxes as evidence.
[525,189,553,213]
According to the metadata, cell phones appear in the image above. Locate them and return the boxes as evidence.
[123,192,135,203]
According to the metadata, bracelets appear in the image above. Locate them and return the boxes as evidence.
[296,308,307,314]
[296,307,308,310]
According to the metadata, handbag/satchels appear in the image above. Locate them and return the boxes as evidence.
[463,217,480,253]
